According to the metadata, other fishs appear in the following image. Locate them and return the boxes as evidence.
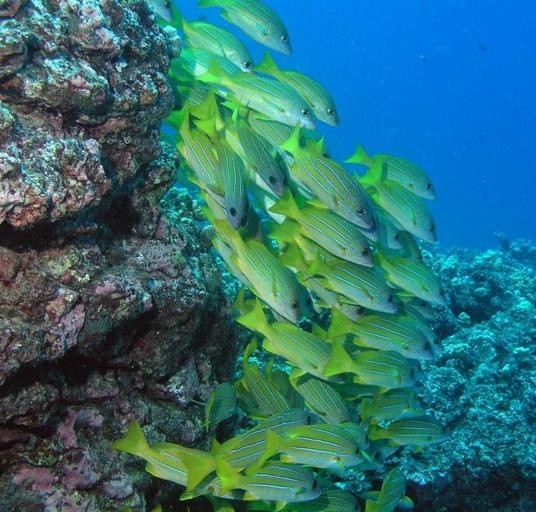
[113,1,453,511]
